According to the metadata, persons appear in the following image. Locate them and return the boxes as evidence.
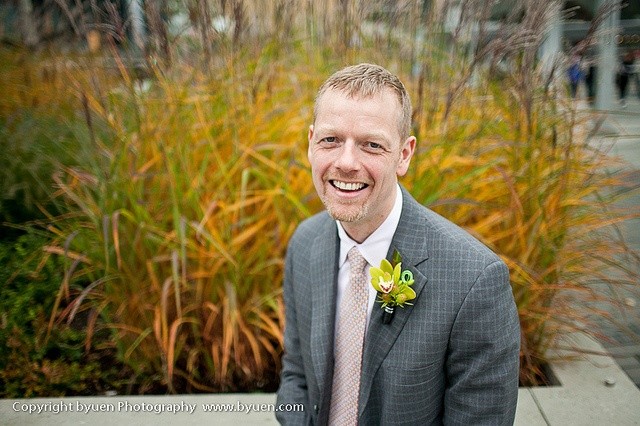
[272,63,522,426]
[565,60,582,102]
[582,62,597,106]
[614,63,629,107]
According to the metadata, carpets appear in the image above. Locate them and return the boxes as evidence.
[328,245,368,426]
